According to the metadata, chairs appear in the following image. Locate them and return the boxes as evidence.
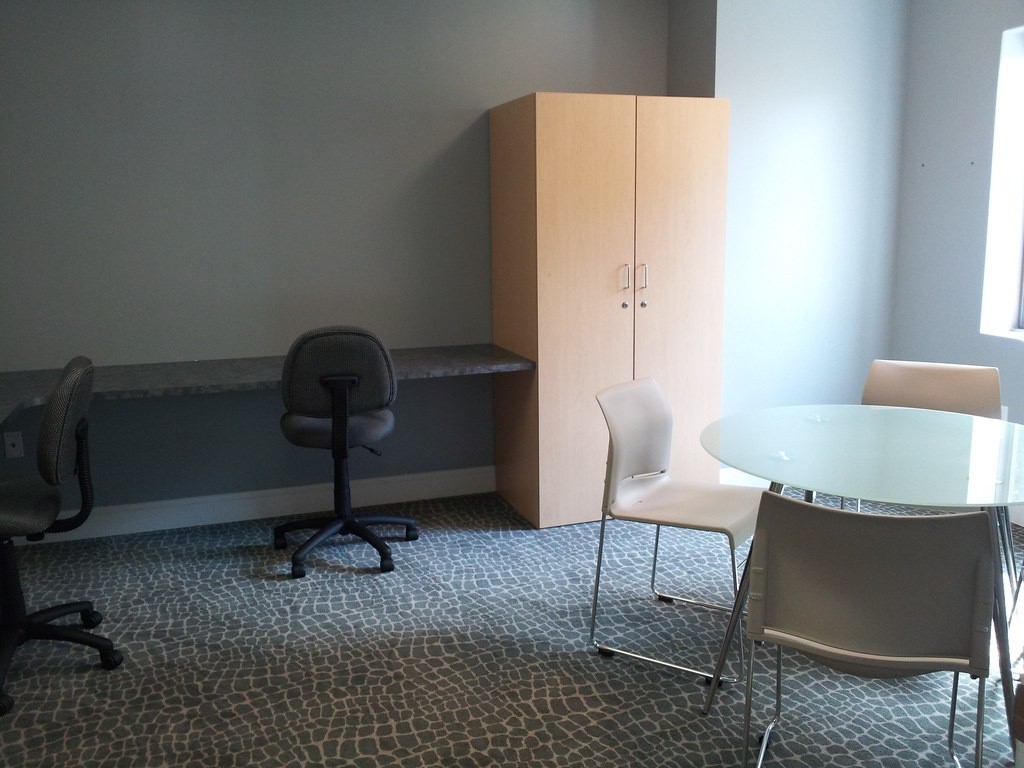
[0,353,124,716]
[272,326,421,579]
[743,490,998,768]
[840,359,1004,521]
[590,376,764,686]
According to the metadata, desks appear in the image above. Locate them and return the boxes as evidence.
[702,406,1023,734]
[0,344,536,419]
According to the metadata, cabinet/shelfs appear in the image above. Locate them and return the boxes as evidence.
[487,92,733,529]
